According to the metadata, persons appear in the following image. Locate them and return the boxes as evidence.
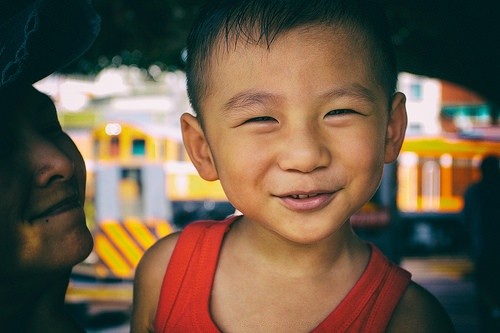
[464,154,500,333]
[0,0,103,332]
[130,0,456,333]
